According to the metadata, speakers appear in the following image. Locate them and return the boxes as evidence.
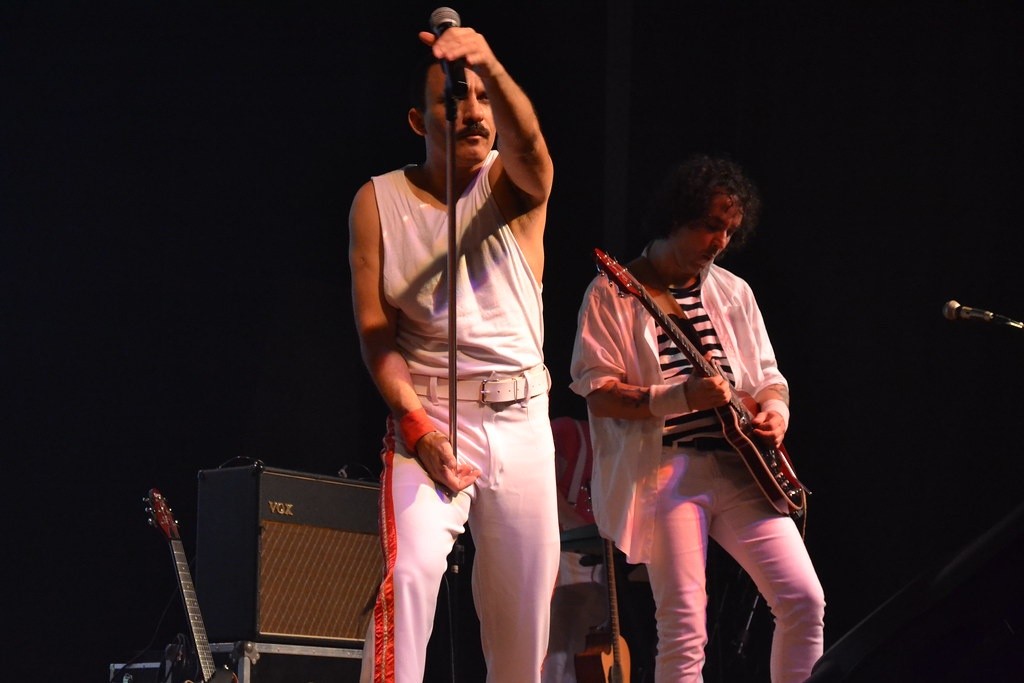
[803,498,1024,683]
[197,459,388,650]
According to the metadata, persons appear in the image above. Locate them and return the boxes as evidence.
[570,154,827,683]
[348,25,562,683]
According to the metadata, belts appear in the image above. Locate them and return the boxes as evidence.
[663,437,732,451]
[410,368,548,404]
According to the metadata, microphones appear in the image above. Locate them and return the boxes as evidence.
[430,7,468,100]
[941,301,994,319]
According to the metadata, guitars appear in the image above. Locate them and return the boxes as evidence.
[592,246,807,518]
[141,486,239,683]
[574,525,631,683]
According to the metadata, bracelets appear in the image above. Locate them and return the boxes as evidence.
[761,400,790,432]
[649,383,691,416]
[398,407,436,453]
